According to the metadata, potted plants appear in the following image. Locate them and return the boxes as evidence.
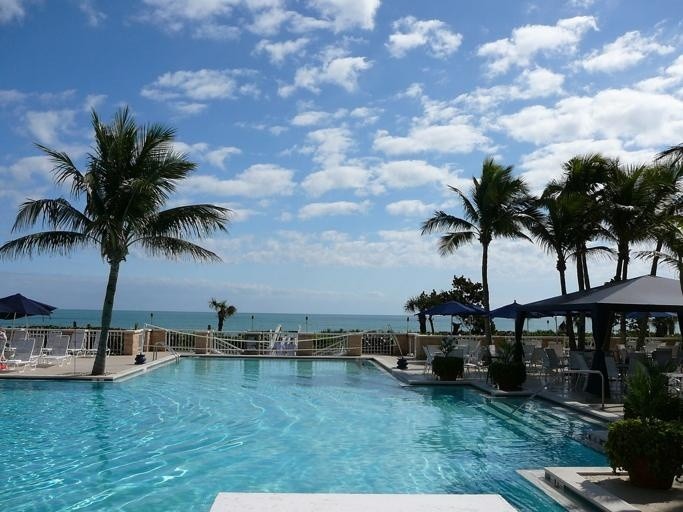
[603,417,683,493]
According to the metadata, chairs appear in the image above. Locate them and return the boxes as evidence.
[420,339,682,402]
[0,323,114,376]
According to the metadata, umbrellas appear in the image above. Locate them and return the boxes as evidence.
[626,312,677,342]
[413,300,478,338]
[490,300,544,319]
[538,311,591,343]
[0,330,7,361]
[462,304,491,340]
[25,299,58,335]
[0,293,51,341]
